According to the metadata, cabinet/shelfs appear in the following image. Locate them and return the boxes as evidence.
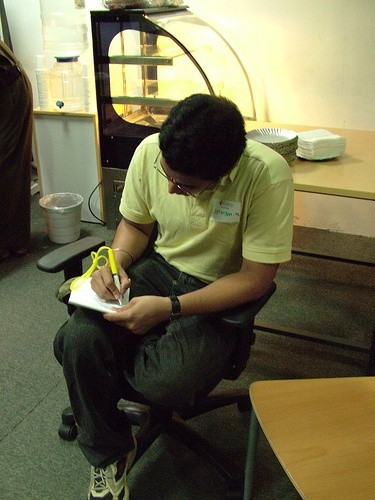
[90,6,256,138]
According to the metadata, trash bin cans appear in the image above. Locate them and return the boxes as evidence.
[39,192,84,245]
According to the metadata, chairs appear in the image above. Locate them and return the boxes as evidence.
[36,236,276,500]
[242,375,375,500]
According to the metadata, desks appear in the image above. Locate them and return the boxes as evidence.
[241,122,375,373]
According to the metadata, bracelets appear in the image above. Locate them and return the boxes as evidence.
[169,295,182,320]
[111,248,135,265]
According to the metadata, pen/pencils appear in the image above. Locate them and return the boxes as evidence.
[108,249,123,305]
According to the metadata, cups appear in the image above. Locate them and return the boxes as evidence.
[34,54,50,110]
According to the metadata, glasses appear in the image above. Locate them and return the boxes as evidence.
[153,150,210,200]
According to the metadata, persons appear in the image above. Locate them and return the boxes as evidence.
[53,93,294,500]
[0,41,33,259]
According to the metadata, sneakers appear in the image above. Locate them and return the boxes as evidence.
[87,432,137,500]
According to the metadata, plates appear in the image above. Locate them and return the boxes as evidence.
[244,127,298,165]
[295,152,335,161]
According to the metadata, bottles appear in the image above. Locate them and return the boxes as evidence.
[49,55,85,112]
